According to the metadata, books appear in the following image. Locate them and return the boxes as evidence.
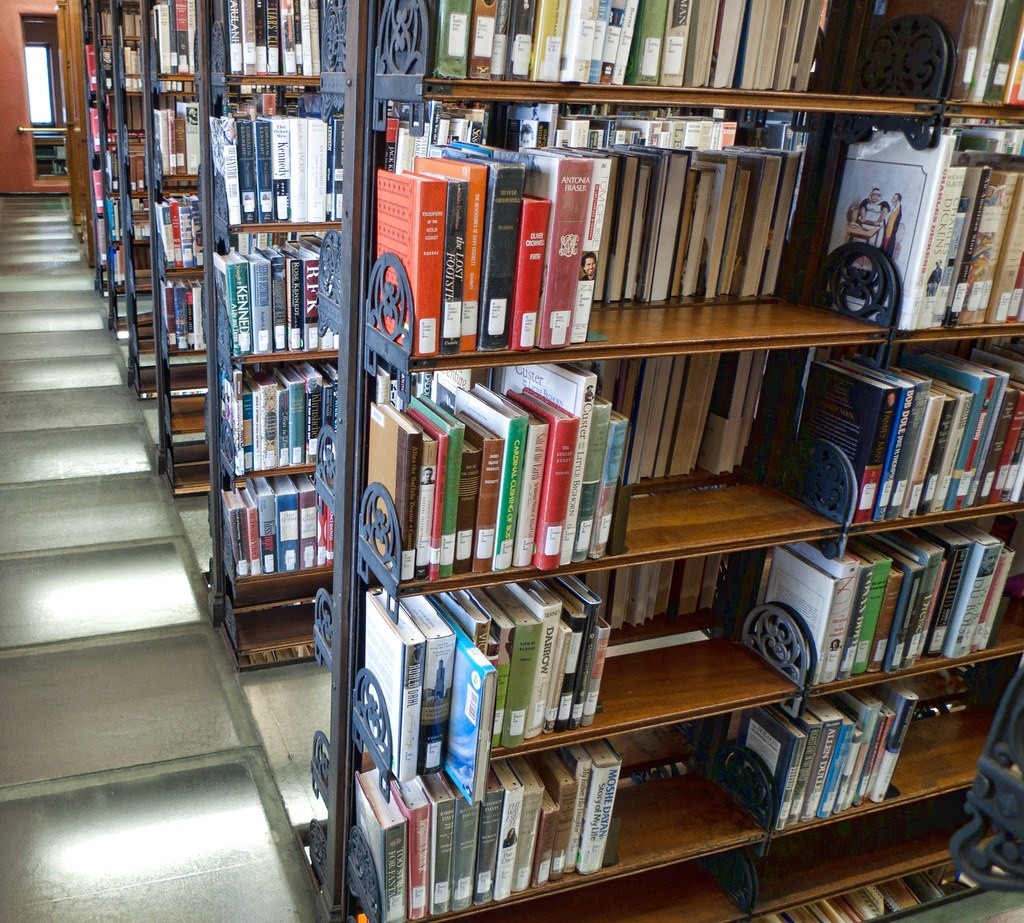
[82,0,1023,922]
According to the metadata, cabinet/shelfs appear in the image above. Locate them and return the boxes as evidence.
[77,0,1024,923]
